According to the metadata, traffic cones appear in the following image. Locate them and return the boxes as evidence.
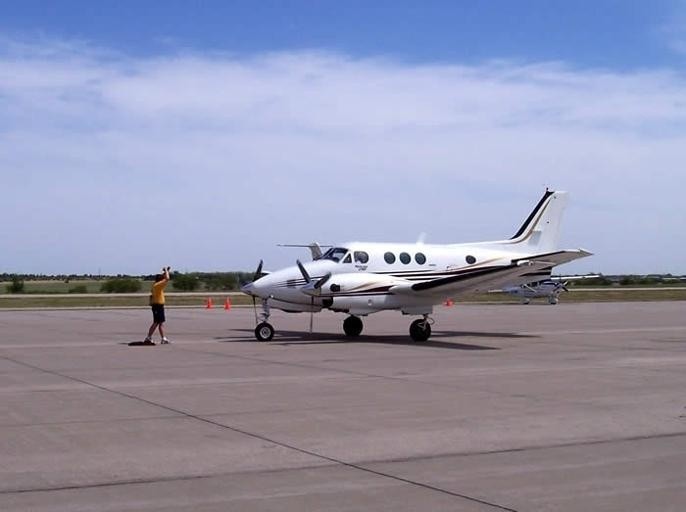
[224,297,231,310]
[207,297,212,309]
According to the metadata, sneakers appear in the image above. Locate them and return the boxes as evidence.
[144,337,156,345]
[161,337,171,344]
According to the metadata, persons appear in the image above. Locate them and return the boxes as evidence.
[143,266,171,345]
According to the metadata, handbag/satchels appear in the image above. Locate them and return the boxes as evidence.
[149,295,153,306]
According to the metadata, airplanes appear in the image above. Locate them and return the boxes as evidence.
[489,275,601,305]
[445,299,454,307]
[241,189,595,344]
